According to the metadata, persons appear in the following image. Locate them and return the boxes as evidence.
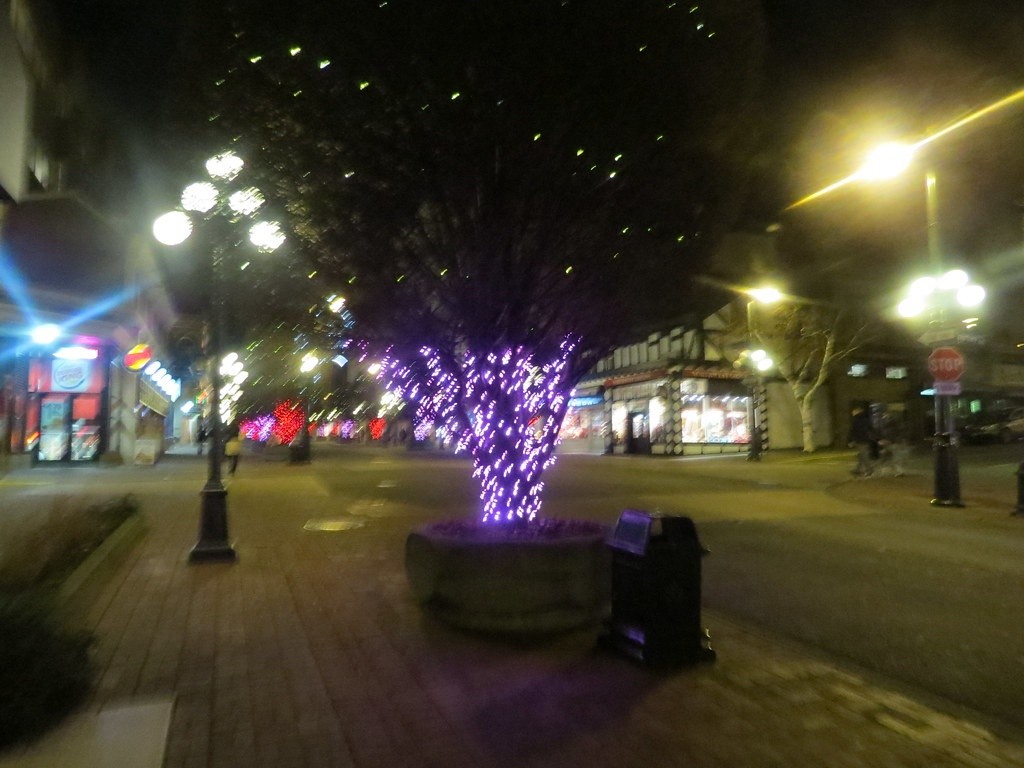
[196,426,214,456]
[878,424,908,478]
[846,400,885,477]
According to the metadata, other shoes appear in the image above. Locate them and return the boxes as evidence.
[864,469,873,478]
[849,466,860,474]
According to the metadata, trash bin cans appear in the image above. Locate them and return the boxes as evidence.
[1010,461,1024,516]
[288,441,304,466]
[930,430,965,506]
[599,509,715,673]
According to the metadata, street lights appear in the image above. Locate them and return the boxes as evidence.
[745,285,781,462]
[846,138,966,507]
[150,147,287,563]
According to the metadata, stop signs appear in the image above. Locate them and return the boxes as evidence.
[928,346,970,387]
